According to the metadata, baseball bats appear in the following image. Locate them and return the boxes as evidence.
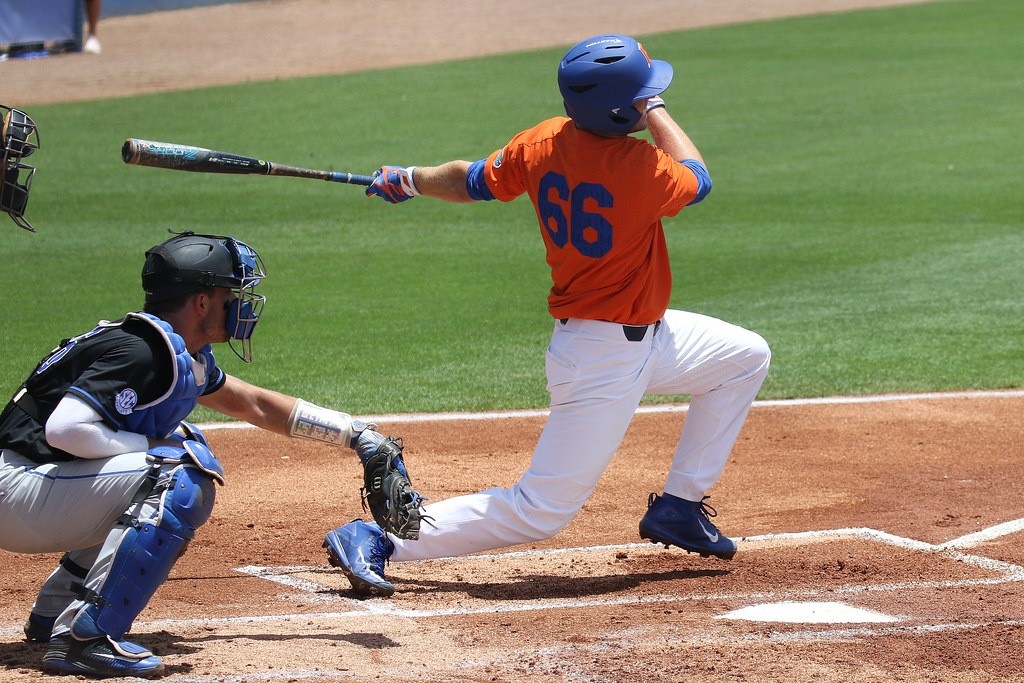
[121,137,378,187]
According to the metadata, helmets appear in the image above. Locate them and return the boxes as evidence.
[558,34,674,132]
[141,227,267,363]
[0,104,40,233]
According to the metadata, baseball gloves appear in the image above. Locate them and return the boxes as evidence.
[363,430,423,541]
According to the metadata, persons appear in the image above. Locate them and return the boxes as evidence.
[78,1,102,54]
[320,33,772,599]
[0,232,437,679]
[0,103,44,237]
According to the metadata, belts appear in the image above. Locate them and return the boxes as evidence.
[560,318,661,341]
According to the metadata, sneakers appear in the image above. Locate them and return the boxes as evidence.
[322,518,395,599]
[43,634,165,678]
[24,612,59,642]
[639,492,737,560]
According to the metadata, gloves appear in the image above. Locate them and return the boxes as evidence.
[647,95,666,112]
[366,165,421,205]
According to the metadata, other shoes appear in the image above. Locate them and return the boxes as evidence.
[84,36,101,54]
